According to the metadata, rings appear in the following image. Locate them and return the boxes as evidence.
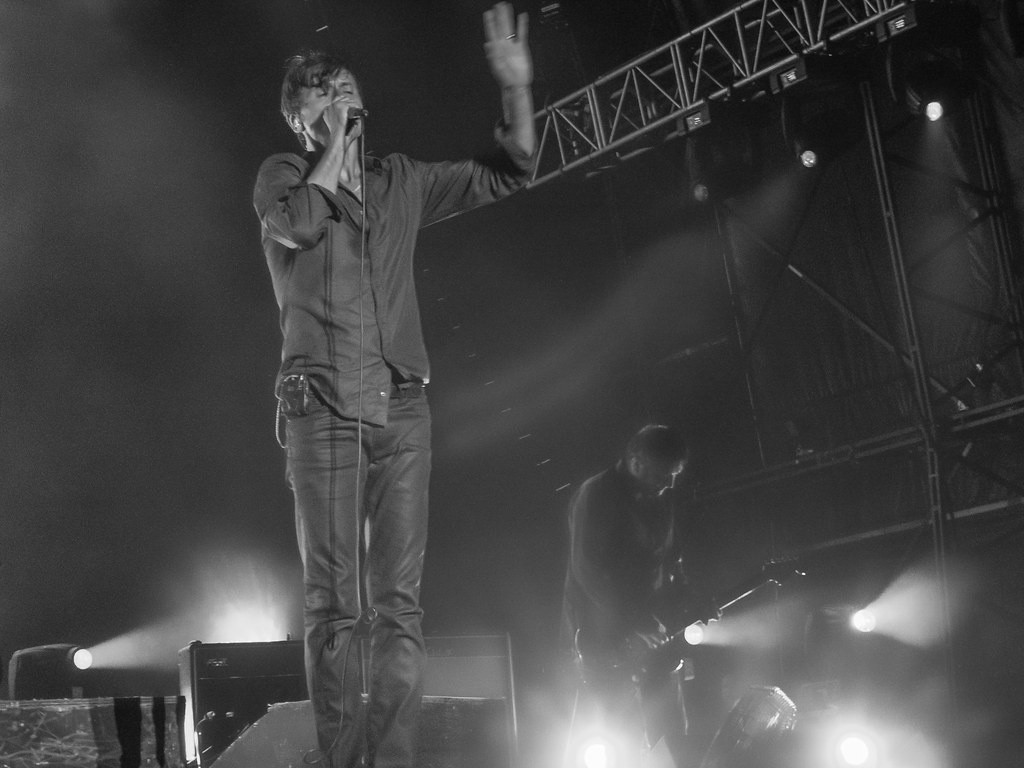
[506,34,516,39]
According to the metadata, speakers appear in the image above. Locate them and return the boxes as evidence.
[359,636,517,741]
[362,694,514,768]
[177,639,324,768]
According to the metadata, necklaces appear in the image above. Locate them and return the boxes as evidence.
[352,185,360,191]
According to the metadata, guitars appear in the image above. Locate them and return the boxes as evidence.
[574,552,812,687]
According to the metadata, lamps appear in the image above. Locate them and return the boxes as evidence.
[925,91,951,122]
[801,143,824,169]
[693,175,717,201]
[674,624,703,649]
[59,646,93,675]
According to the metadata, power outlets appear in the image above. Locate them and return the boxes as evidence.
[71,687,83,699]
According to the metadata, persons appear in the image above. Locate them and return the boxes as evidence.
[255,2,540,767]
[562,425,722,756]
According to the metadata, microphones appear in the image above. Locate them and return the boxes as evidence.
[346,107,368,119]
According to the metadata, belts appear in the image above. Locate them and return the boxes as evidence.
[389,385,422,398]
